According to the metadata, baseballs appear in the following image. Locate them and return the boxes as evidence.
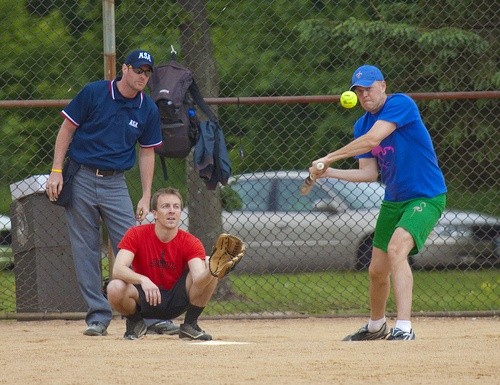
[340,91,358,109]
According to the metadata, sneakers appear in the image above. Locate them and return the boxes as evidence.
[123,320,148,339]
[342,322,389,342]
[380,328,415,342]
[83,322,108,337]
[144,317,181,335]
[179,323,213,341]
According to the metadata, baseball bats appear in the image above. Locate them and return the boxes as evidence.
[298,161,325,195]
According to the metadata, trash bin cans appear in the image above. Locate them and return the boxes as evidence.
[8,176,103,321]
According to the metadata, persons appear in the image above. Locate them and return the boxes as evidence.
[309,65,447,341]
[108,187,245,341]
[46,50,164,336]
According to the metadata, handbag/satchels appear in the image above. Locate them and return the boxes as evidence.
[43,161,81,206]
[144,54,218,181]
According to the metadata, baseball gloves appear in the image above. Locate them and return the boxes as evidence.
[208,233,246,279]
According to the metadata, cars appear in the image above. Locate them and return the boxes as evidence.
[136,169,500,276]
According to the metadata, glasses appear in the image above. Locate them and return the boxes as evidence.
[127,65,152,77]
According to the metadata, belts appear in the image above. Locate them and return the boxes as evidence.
[79,164,124,177]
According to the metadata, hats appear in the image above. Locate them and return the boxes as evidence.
[125,49,155,73]
[348,65,384,91]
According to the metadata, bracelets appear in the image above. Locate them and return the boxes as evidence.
[51,168,63,173]
